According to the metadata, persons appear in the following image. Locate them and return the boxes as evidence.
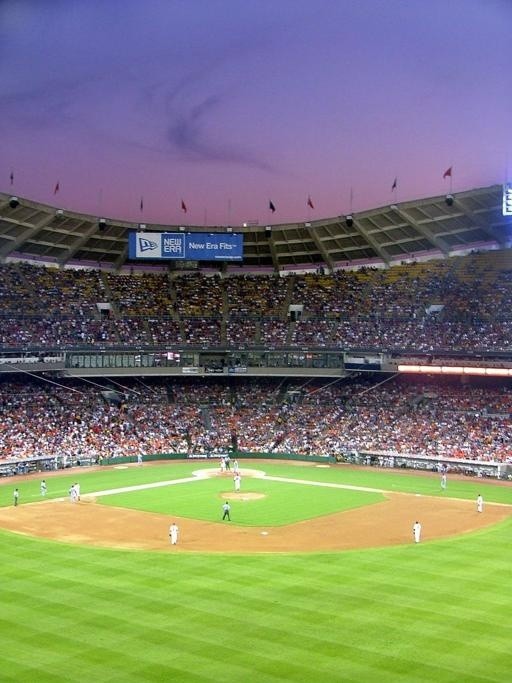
[41,479,47,497]
[233,472,241,491]
[169,522,178,544]
[74,482,81,502]
[477,493,482,512]
[221,501,231,520]
[14,488,19,505]
[412,521,421,542]
[1,259,512,474]
[441,472,446,489]
[70,485,77,503]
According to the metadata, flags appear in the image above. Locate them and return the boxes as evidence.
[181,199,188,213]
[307,197,313,208]
[392,178,396,190]
[443,166,451,178]
[53,181,61,194]
[269,199,276,213]
